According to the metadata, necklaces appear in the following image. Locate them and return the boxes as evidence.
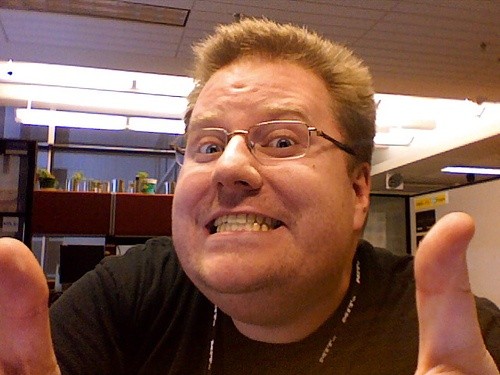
[206,255,361,375]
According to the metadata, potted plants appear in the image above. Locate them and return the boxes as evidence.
[37,168,56,191]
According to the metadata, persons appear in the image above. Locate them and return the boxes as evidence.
[0,17,500,375]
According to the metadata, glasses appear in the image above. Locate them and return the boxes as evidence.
[169,120,357,167]
